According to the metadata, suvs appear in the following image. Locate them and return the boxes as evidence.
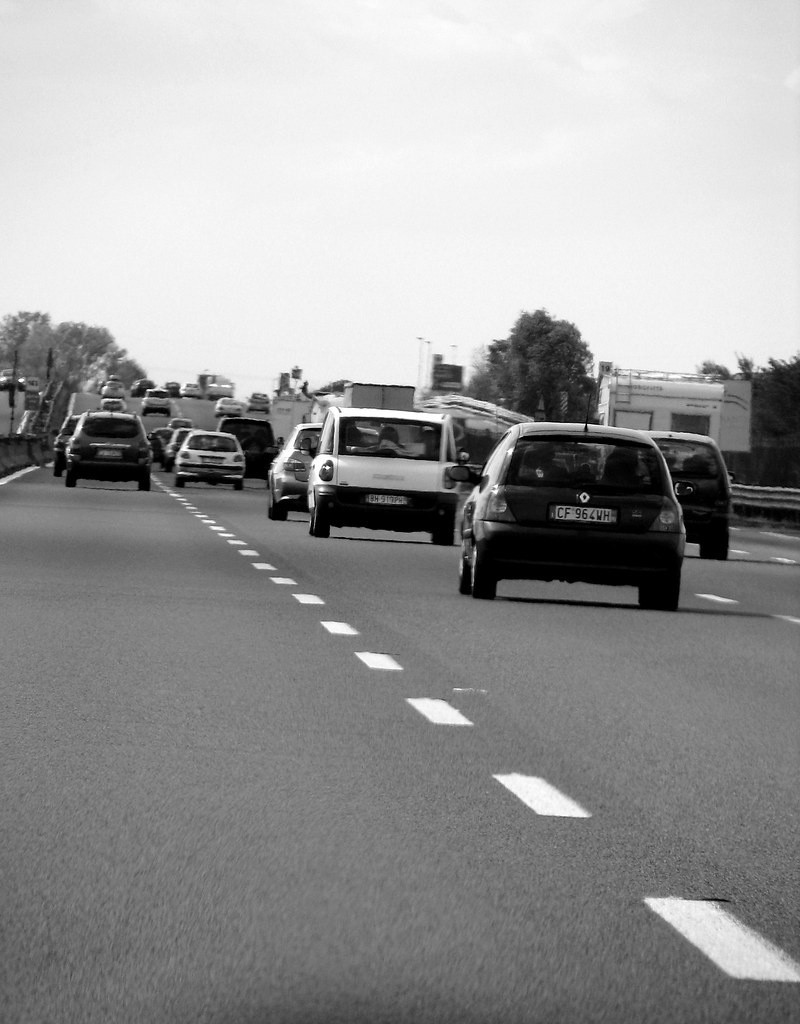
[65,409,154,491]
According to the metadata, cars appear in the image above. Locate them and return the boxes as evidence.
[268,423,379,521]
[141,388,171,417]
[215,398,243,418]
[149,416,194,473]
[171,430,246,490]
[308,406,471,546]
[218,417,285,489]
[165,382,180,397]
[130,379,155,398]
[179,382,201,400]
[245,393,271,414]
[640,430,736,561]
[446,422,686,610]
[101,381,125,400]
[52,415,81,477]
[96,397,127,413]
[0,368,27,390]
